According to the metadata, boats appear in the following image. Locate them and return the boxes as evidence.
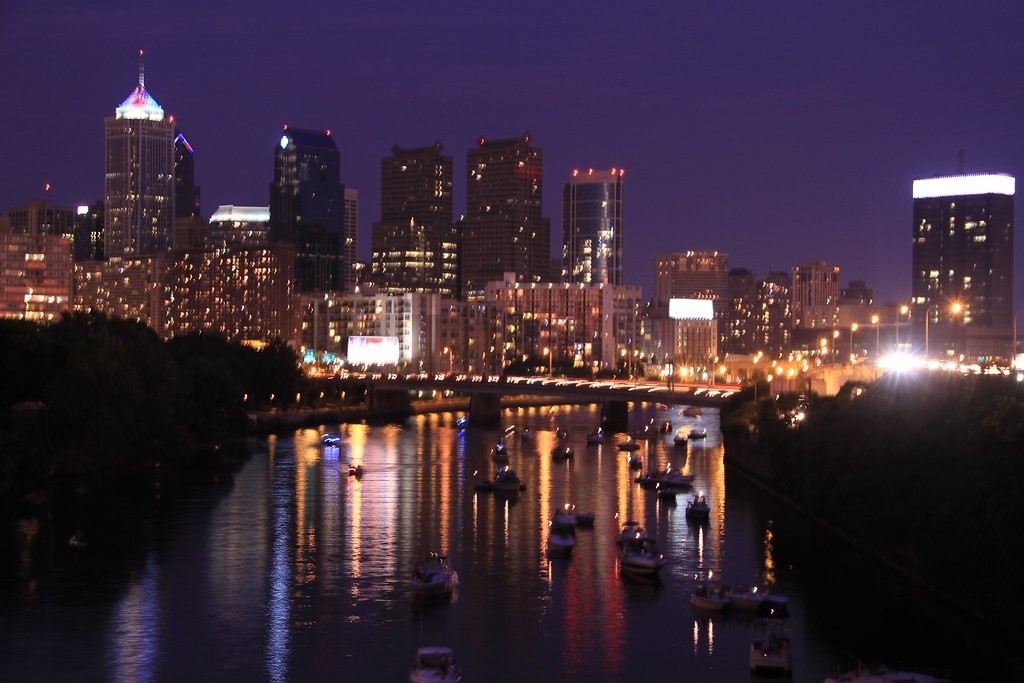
[314,399,943,683]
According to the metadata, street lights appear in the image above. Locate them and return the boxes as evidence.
[662,298,971,395]
